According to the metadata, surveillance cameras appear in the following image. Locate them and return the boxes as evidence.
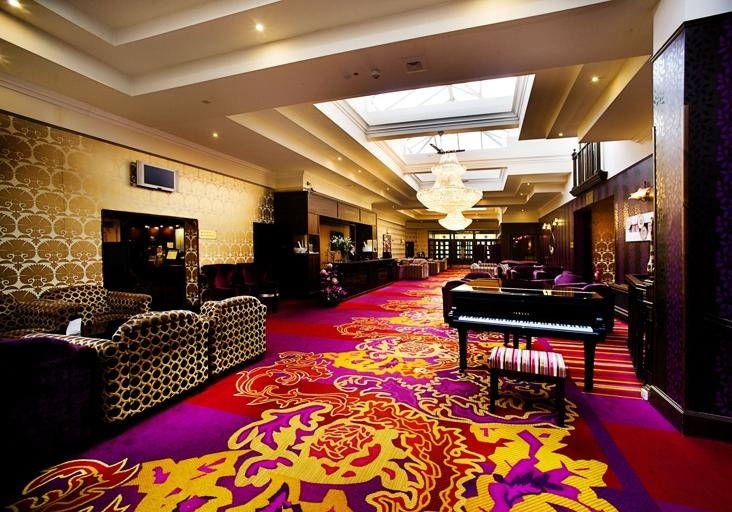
[370,70,382,81]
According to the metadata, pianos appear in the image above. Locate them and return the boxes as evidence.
[448,284,615,393]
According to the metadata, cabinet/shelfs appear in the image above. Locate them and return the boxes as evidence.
[274,191,377,296]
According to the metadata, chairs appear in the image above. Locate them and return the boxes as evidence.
[461,260,587,286]
[1,285,267,424]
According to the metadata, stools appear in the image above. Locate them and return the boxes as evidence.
[489,346,566,427]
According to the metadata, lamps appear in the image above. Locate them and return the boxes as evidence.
[416,133,484,214]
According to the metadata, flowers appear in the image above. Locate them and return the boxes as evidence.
[318,262,348,300]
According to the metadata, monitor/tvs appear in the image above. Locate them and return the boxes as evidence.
[136,160,176,192]
